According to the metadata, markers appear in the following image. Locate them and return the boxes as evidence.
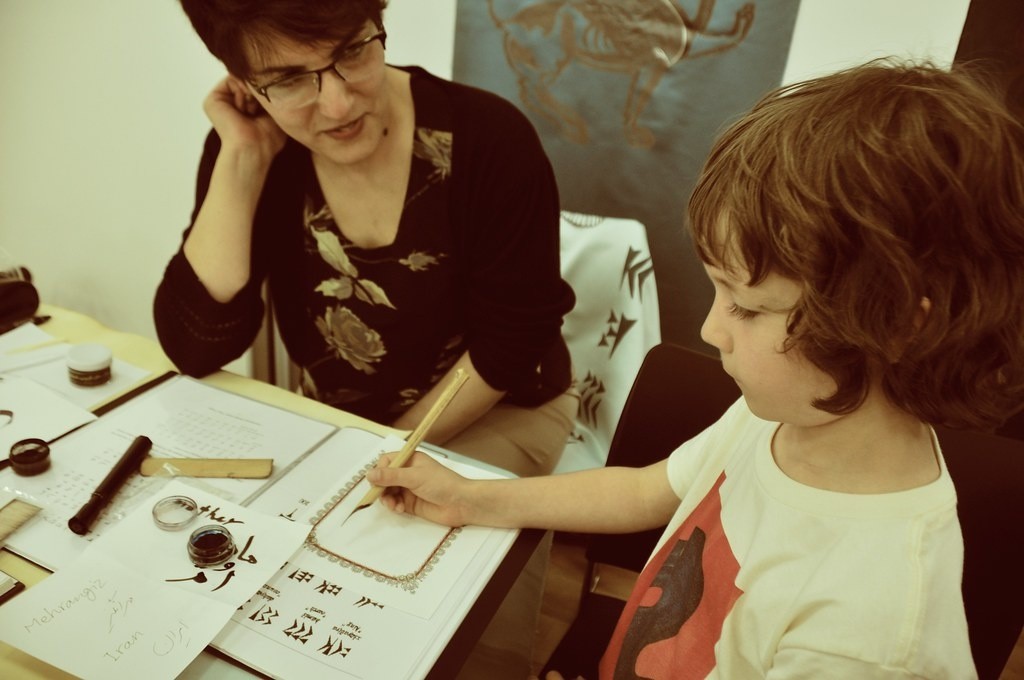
[68,436,152,536]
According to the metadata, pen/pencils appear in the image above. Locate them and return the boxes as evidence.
[341,367,471,528]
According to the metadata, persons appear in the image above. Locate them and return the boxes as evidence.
[367,62,1024,680]
[152,0,580,476]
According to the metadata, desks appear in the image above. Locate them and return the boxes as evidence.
[0,307,546,680]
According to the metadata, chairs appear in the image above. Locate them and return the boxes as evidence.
[250,208,666,474]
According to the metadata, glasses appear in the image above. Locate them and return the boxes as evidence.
[248,30,389,110]
